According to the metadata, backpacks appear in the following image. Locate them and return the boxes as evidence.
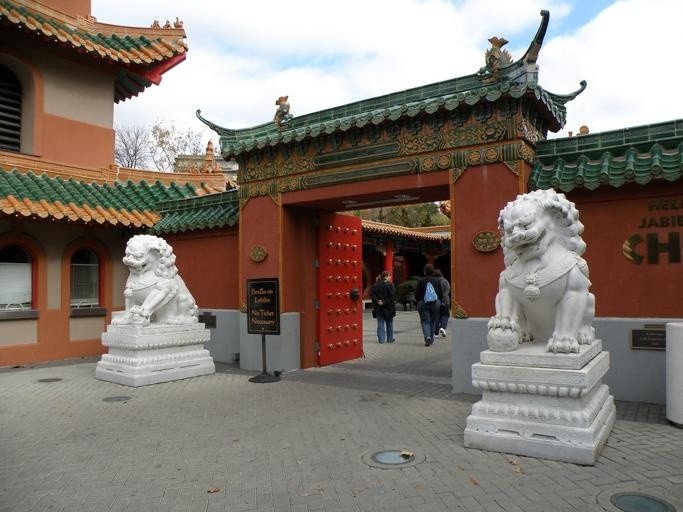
[422,277,438,306]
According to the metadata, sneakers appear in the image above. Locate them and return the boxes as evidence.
[425,328,447,346]
[387,339,396,343]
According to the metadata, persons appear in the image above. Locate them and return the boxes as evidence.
[367,270,396,344]
[432,267,450,340]
[413,264,442,346]
